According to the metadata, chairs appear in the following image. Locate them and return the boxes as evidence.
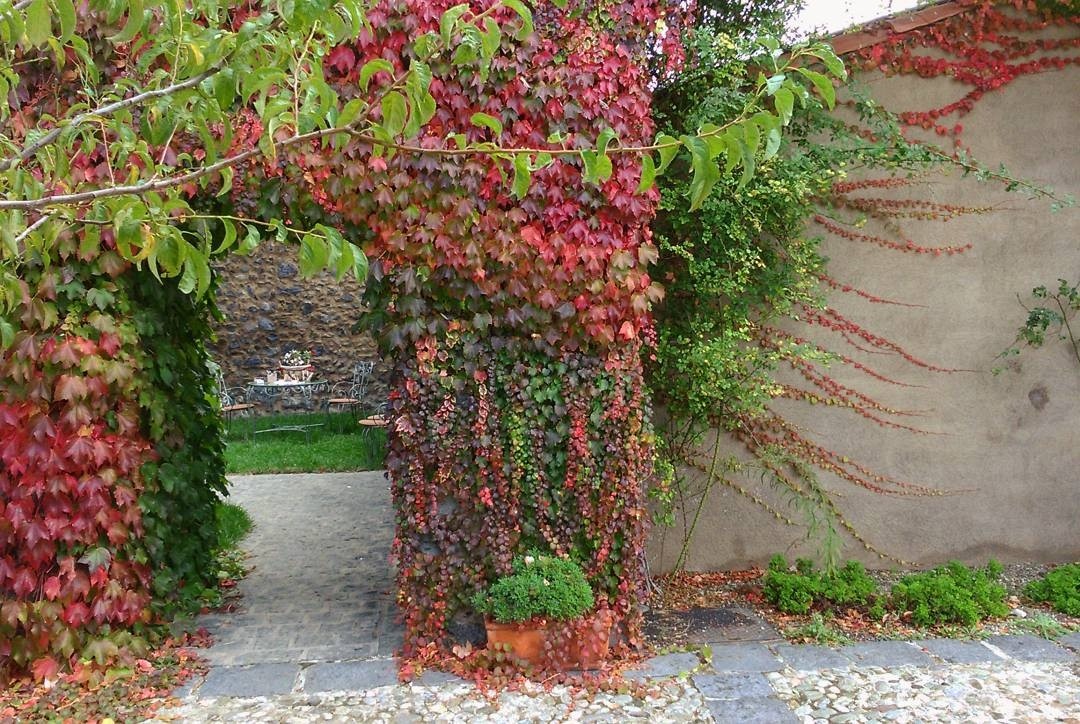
[326,360,373,434]
[359,402,395,462]
[216,363,254,435]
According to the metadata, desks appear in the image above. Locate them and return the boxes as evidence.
[246,379,329,448]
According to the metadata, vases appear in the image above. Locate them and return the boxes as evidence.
[280,364,312,380]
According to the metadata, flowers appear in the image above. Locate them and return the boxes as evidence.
[280,348,311,366]
